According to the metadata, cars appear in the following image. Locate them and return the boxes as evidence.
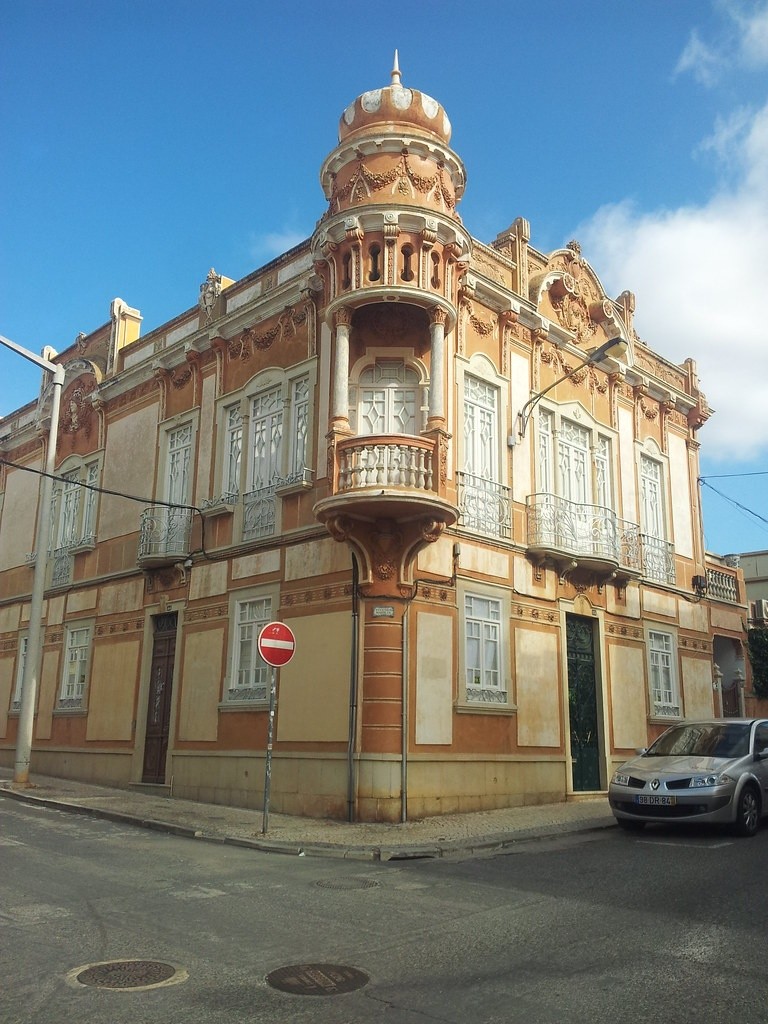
[608,717,768,836]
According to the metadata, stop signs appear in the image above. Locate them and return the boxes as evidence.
[257,619,297,666]
